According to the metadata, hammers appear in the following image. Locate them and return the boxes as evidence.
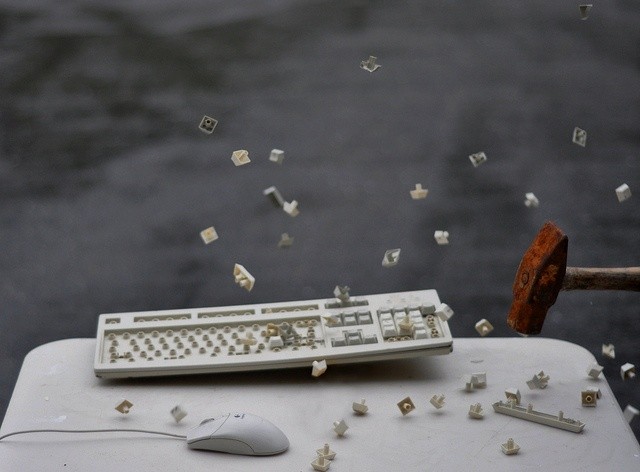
[506,221,640,338]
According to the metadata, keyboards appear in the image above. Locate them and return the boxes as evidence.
[93,287,453,377]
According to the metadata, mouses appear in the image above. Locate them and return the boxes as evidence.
[187,409,291,457]
